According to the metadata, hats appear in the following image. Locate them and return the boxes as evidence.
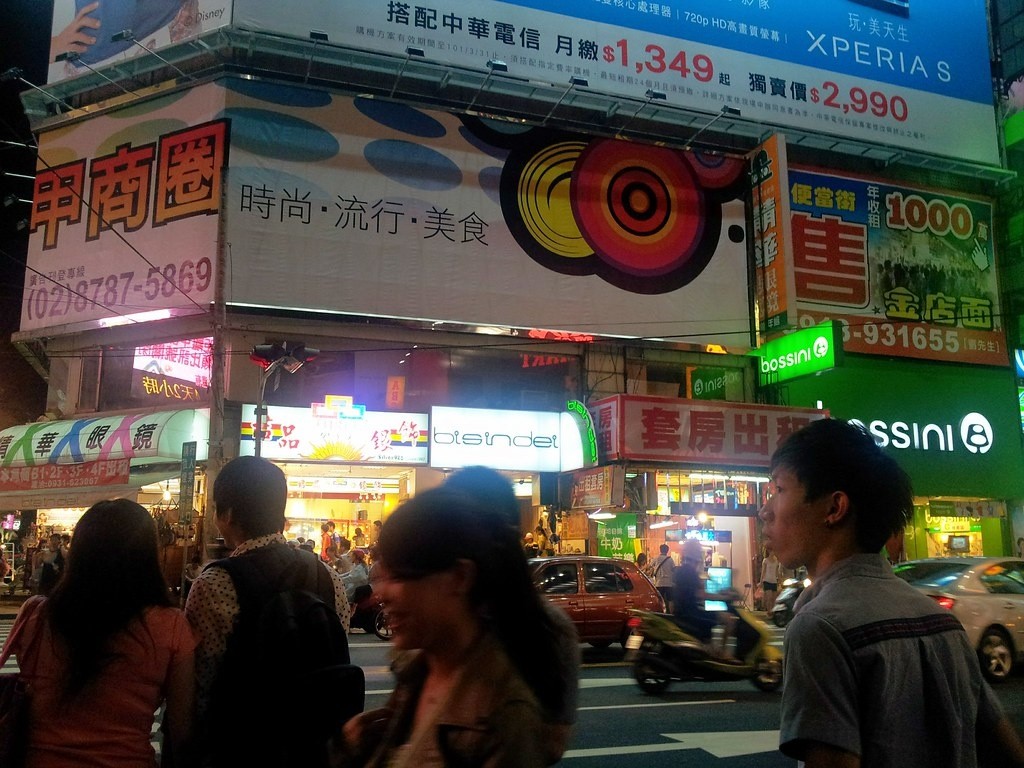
[352,549,367,566]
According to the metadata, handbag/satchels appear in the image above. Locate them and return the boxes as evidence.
[0,594,56,768]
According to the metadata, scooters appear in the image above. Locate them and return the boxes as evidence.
[623,588,785,695]
[772,577,812,627]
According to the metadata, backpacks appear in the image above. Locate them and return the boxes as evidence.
[208,549,365,768]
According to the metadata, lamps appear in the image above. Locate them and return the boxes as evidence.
[110,29,195,83]
[467,58,508,110]
[17,219,31,231]
[0,67,76,112]
[3,194,34,207]
[617,88,666,134]
[304,29,329,84]
[54,51,141,101]
[684,104,741,148]
[390,46,425,97]
[543,76,589,125]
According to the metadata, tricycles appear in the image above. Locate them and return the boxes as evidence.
[345,578,397,640]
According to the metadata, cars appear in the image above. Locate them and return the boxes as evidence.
[889,555,1024,686]
[526,556,666,649]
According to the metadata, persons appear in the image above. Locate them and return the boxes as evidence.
[1017,537,1024,558]
[7,498,195,768]
[343,492,543,768]
[184,455,351,768]
[27,520,552,595]
[441,466,578,738]
[635,537,739,662]
[759,418,1024,768]
[761,545,780,618]
